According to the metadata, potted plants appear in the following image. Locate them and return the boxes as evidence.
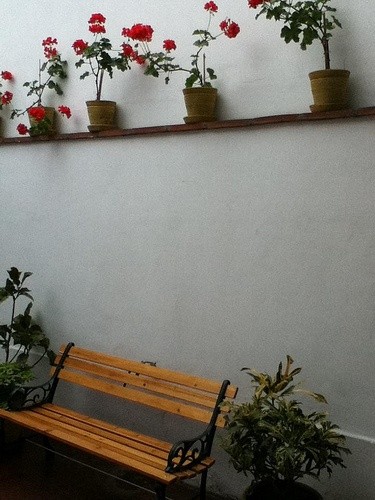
[218,355,351,500]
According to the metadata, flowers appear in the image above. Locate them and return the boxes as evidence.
[248,0,342,70]
[73,14,132,100]
[128,1,241,87]
[2,37,72,136]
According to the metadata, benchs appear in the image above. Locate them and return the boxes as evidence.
[0,342,238,500]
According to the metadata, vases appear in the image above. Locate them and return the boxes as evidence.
[182,86,218,123]
[86,101,118,132]
[27,107,57,138]
[308,69,351,113]
